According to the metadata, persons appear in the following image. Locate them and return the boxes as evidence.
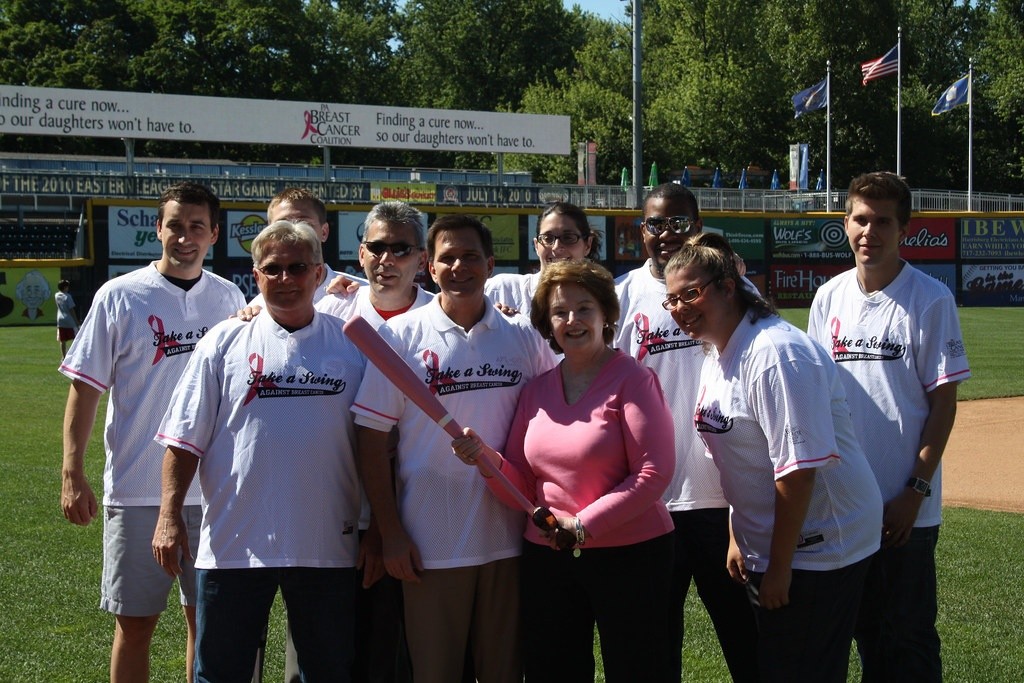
[663,231,884,683]
[228,188,371,321]
[325,201,606,363]
[151,219,396,683]
[606,182,762,683]
[451,258,682,683]
[55,279,80,363]
[58,181,248,683]
[806,171,971,683]
[312,200,521,332]
[348,212,560,683]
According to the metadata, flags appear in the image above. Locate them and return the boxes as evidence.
[931,73,970,116]
[791,76,827,119]
[860,42,898,86]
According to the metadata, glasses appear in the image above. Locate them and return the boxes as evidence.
[364,241,424,258]
[259,261,322,274]
[662,270,728,310]
[531,506,577,551]
[536,232,588,245]
[644,216,697,234]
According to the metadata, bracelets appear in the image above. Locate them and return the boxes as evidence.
[570,517,585,558]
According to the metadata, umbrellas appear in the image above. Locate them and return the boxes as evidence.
[619,161,824,192]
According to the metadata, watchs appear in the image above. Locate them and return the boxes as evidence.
[905,476,931,498]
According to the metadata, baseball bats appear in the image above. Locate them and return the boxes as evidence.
[340,314,561,551]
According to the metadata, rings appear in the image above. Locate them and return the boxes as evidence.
[884,530,891,535]
[468,456,475,462]
[462,453,467,458]
